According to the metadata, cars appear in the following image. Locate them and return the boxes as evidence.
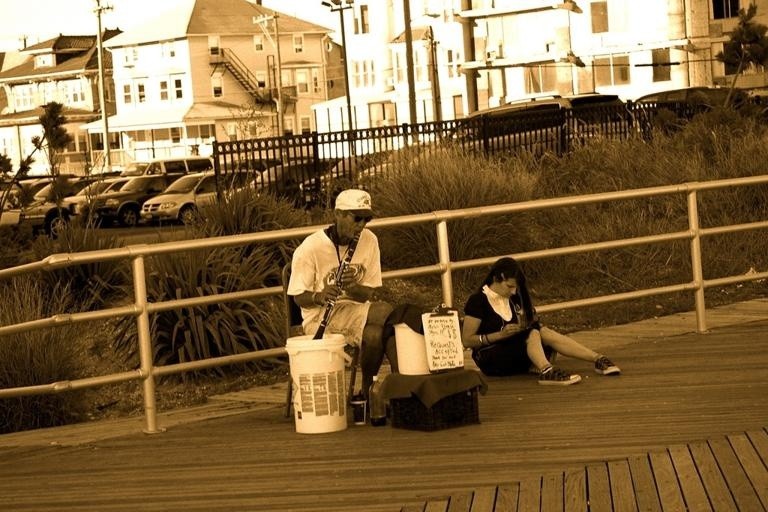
[138,169,261,227]
[0,188,71,241]
[225,157,414,209]
[0,156,215,226]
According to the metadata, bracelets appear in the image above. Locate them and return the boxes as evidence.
[310,291,319,305]
[478,334,492,346]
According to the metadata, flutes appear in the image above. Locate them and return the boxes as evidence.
[312,233,361,340]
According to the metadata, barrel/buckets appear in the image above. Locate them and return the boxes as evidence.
[283,335,350,433]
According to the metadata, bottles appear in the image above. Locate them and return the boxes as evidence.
[368,375,386,427]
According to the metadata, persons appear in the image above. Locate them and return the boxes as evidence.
[283,187,403,412]
[459,255,622,386]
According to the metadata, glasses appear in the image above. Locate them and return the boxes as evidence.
[348,211,373,222]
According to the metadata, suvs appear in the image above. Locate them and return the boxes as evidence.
[409,90,643,173]
[626,82,753,136]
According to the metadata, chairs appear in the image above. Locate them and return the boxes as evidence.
[281,260,362,418]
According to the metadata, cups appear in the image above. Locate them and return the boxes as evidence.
[351,394,367,425]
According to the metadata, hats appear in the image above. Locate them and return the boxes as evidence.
[335,189,372,210]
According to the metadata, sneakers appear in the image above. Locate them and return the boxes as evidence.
[593,353,621,375]
[538,366,581,386]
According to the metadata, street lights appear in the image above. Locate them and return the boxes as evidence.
[318,0,360,161]
[400,10,442,145]
